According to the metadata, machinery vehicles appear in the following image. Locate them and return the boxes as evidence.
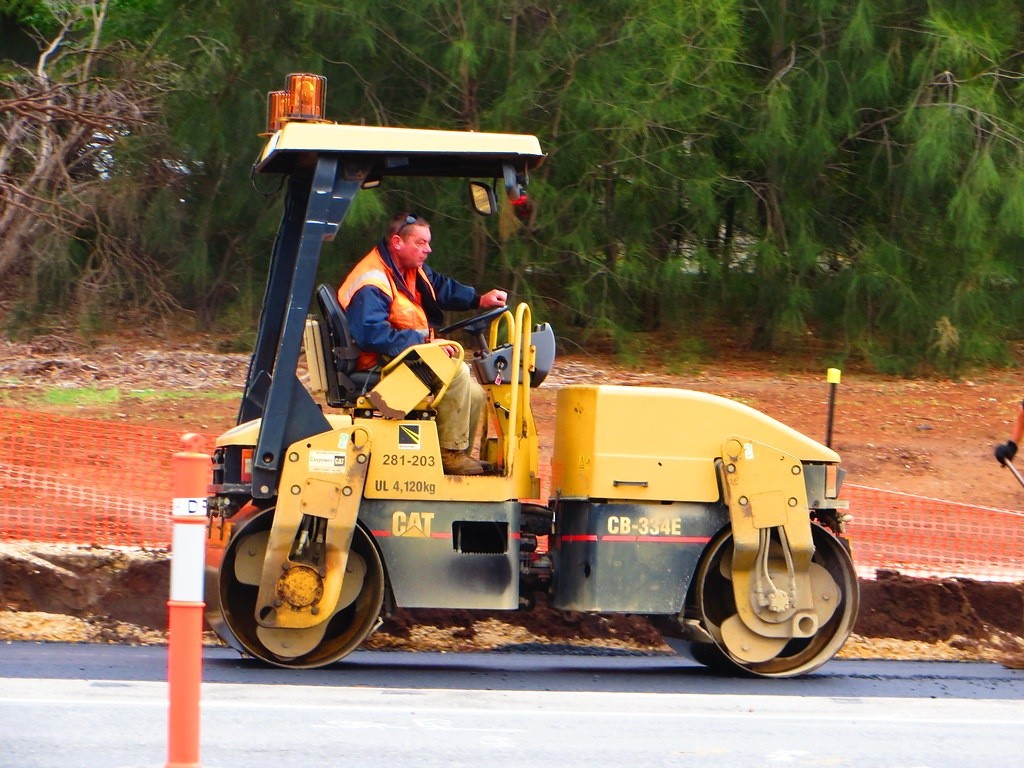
[206,71,862,680]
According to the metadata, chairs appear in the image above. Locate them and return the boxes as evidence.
[315,284,380,390]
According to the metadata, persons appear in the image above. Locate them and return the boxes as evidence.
[993,399,1024,469]
[337,213,508,475]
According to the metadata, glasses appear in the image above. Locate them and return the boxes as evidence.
[396,211,422,234]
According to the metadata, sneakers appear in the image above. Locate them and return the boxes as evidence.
[441,449,492,474]
